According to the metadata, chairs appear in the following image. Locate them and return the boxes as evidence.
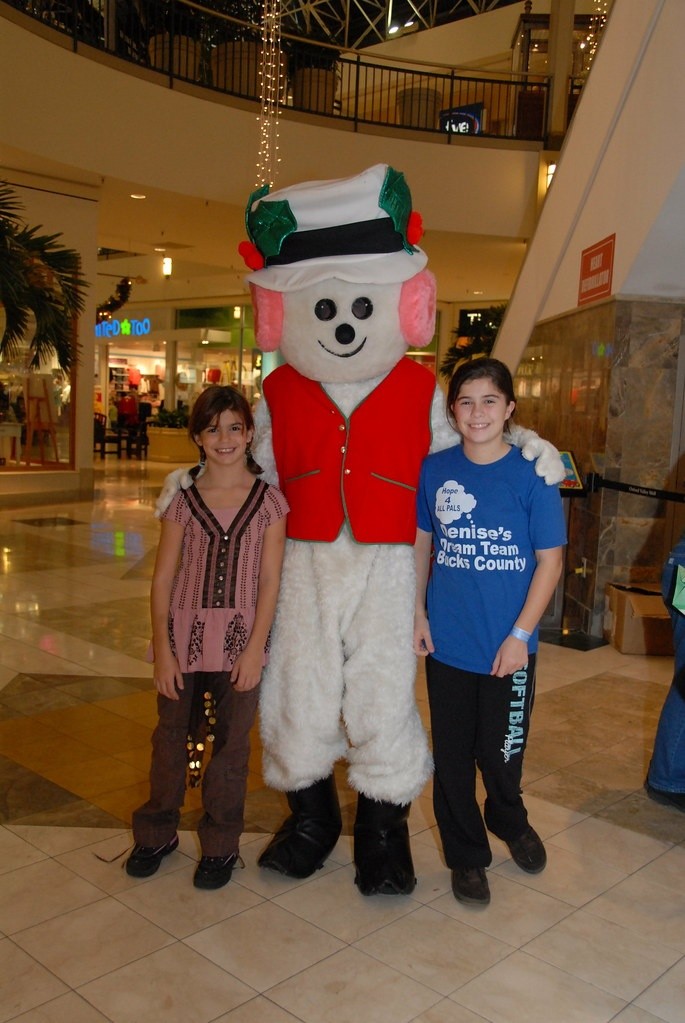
[94,413,148,458]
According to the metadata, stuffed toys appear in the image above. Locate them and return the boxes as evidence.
[153,160,565,898]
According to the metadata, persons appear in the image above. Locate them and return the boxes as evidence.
[644,537,685,815]
[125,382,290,889]
[414,357,567,911]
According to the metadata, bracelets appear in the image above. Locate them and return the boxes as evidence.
[509,625,532,643]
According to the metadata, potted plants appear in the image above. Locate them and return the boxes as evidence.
[290,30,341,116]
[144,1,202,85]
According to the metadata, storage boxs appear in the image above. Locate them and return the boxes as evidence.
[605,581,675,659]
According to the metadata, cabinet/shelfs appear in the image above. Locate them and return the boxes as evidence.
[111,373,131,392]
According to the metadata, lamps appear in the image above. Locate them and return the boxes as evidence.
[384,25,402,39]
[401,22,419,34]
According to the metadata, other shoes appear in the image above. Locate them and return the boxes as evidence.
[451,867,490,905]
[507,823,547,873]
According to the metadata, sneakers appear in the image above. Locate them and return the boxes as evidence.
[194,847,239,888]
[646,780,685,812]
[126,831,179,876]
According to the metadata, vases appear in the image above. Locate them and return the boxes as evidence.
[394,87,444,128]
[210,40,290,103]
